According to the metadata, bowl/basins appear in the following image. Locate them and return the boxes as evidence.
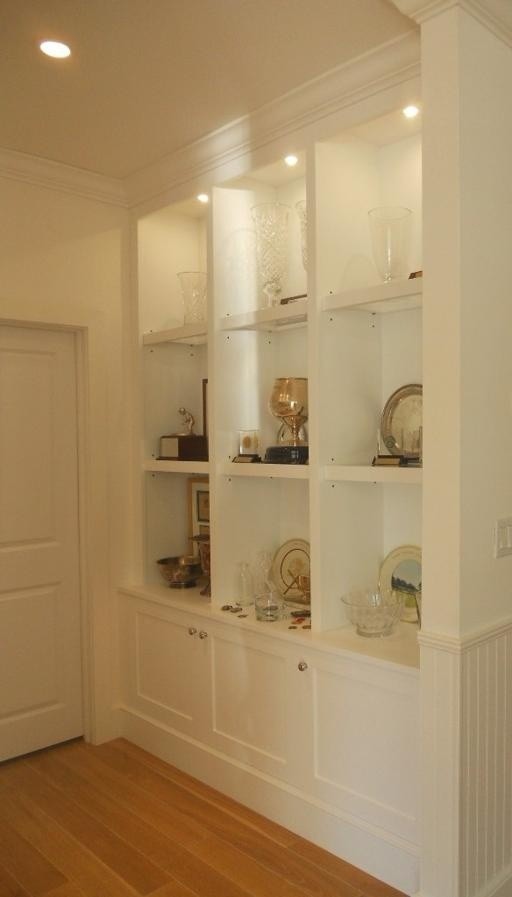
[341,590,407,639]
[156,556,203,589]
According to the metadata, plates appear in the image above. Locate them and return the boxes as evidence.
[380,384,422,463]
[379,545,421,624]
[277,423,306,442]
[272,539,311,600]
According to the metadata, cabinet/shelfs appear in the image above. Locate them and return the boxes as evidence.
[297,639,512,895]
[119,78,423,667]
[123,582,297,836]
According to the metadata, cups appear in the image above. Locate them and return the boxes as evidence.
[269,378,308,446]
[254,591,284,621]
[296,200,308,275]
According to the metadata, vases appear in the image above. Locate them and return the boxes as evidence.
[294,199,309,293]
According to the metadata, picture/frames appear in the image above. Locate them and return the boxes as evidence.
[189,478,210,556]
